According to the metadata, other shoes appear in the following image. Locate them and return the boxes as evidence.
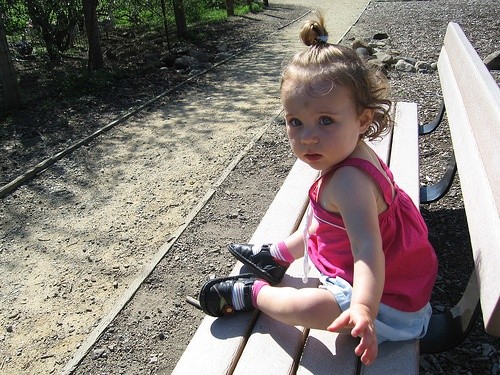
[200,273,270,318]
[229,243,287,284]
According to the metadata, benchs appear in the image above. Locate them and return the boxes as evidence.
[166,21,500,375]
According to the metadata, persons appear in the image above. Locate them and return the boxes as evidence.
[198,10,440,367]
